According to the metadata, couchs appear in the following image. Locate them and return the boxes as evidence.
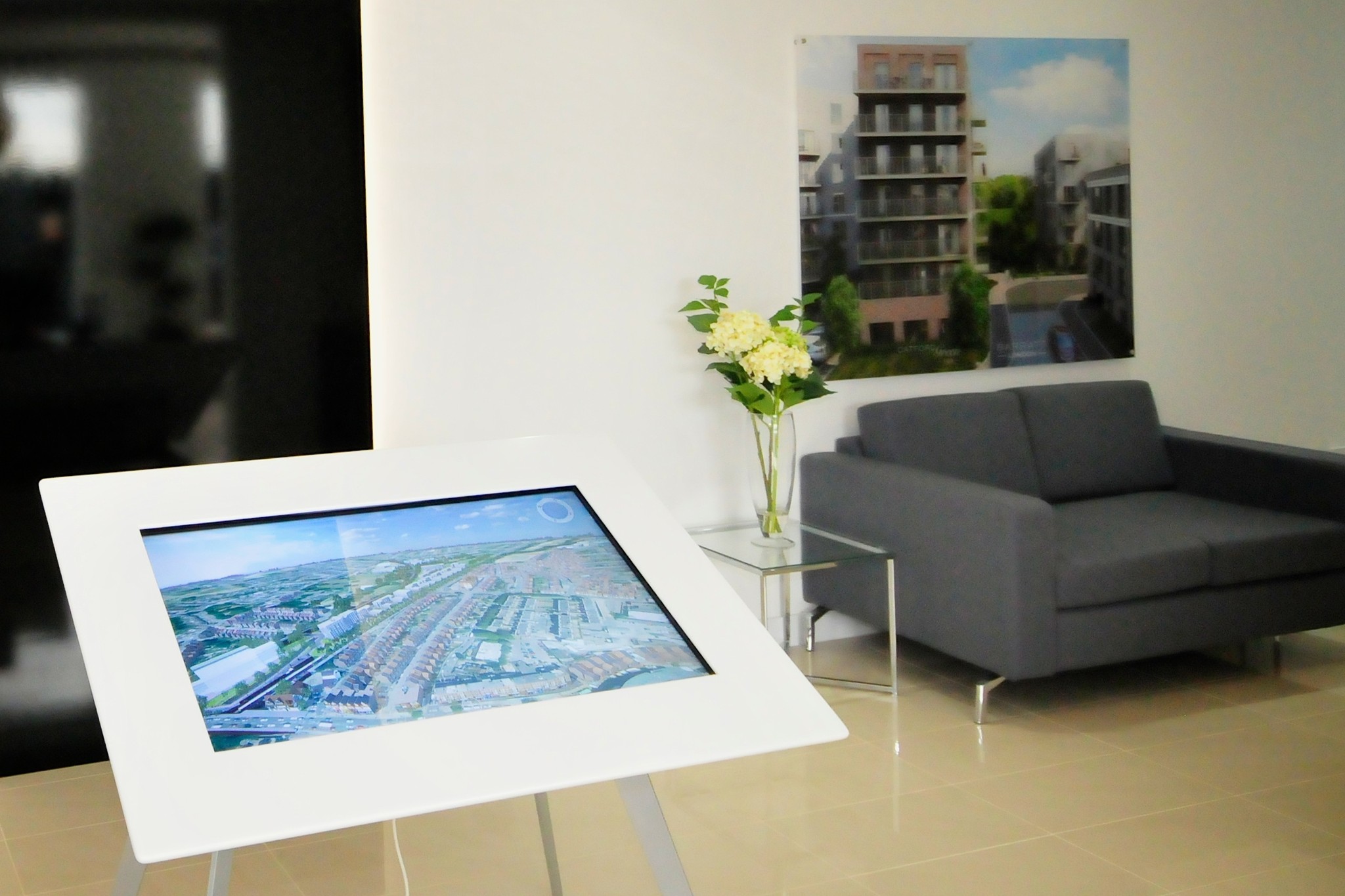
[800,379,1345,724]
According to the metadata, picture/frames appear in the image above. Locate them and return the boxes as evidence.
[42,427,846,869]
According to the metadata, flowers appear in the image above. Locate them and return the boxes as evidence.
[680,272,839,538]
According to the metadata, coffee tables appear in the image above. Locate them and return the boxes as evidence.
[692,521,902,699]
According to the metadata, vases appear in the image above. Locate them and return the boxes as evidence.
[749,409,798,550]
